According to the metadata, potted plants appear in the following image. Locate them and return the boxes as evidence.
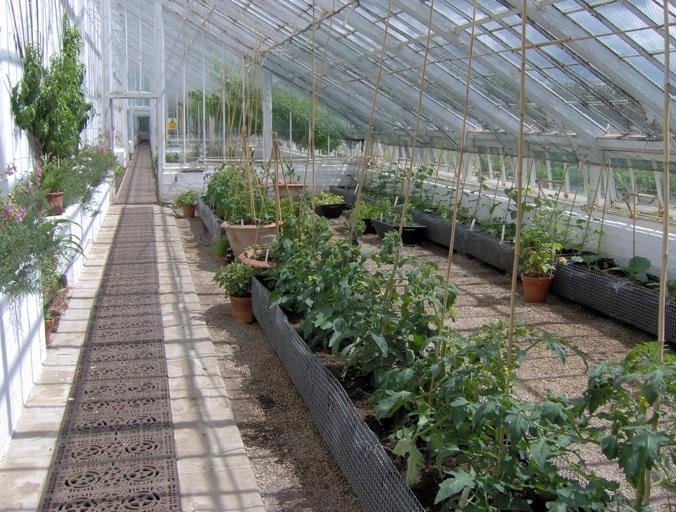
[516,211,563,303]
[309,189,429,247]
[175,190,199,217]
[41,272,68,347]
[213,169,284,324]
[39,164,65,216]
